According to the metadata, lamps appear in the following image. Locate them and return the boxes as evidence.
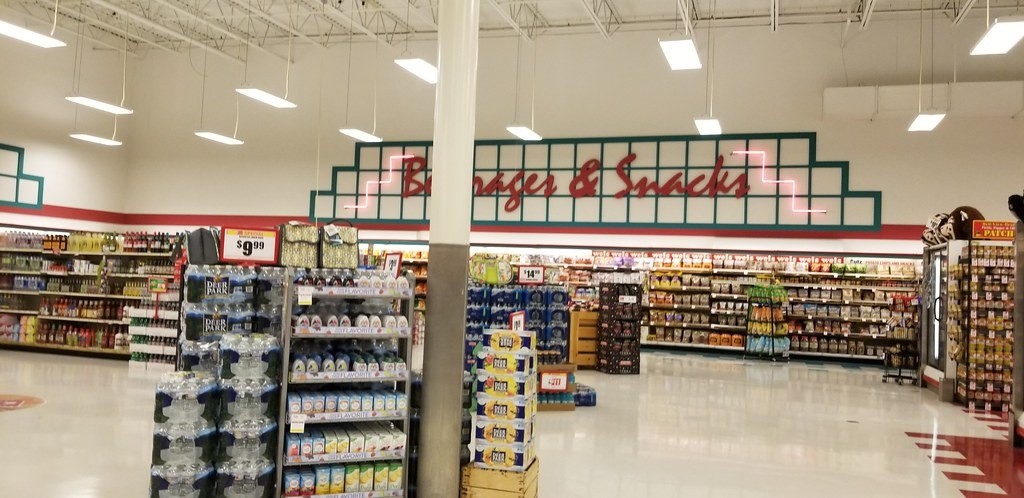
[505,5,543,141]
[69,2,123,147]
[0,0,67,49]
[234,0,298,109]
[394,0,437,85]
[906,0,947,132]
[968,0,1024,55]
[64,1,134,118]
[657,0,703,71]
[339,0,384,142]
[694,0,722,136]
[193,25,244,146]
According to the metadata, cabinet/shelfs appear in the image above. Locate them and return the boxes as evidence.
[0,229,427,498]
[466,240,1024,448]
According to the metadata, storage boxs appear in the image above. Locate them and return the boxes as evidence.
[460,329,539,498]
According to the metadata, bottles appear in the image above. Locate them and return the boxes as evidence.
[0,231,395,497]
